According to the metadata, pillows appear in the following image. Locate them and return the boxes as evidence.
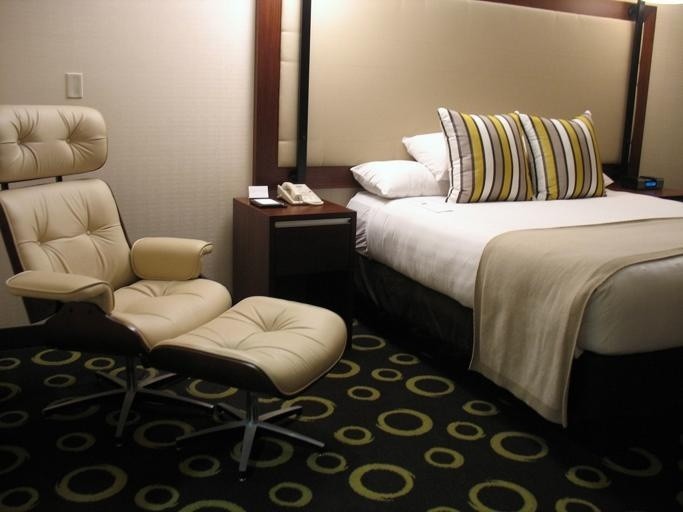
[515,110,608,201]
[402,132,451,182]
[603,171,614,189]
[349,160,448,200]
[436,107,535,202]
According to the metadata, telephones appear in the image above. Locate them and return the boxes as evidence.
[277,182,324,205]
[624,175,664,190]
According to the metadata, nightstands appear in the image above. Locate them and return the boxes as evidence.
[232,197,357,304]
[634,187,683,201]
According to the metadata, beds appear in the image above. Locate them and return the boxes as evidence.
[253,0,682,465]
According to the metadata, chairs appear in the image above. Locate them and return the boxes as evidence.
[0,106,349,483]
